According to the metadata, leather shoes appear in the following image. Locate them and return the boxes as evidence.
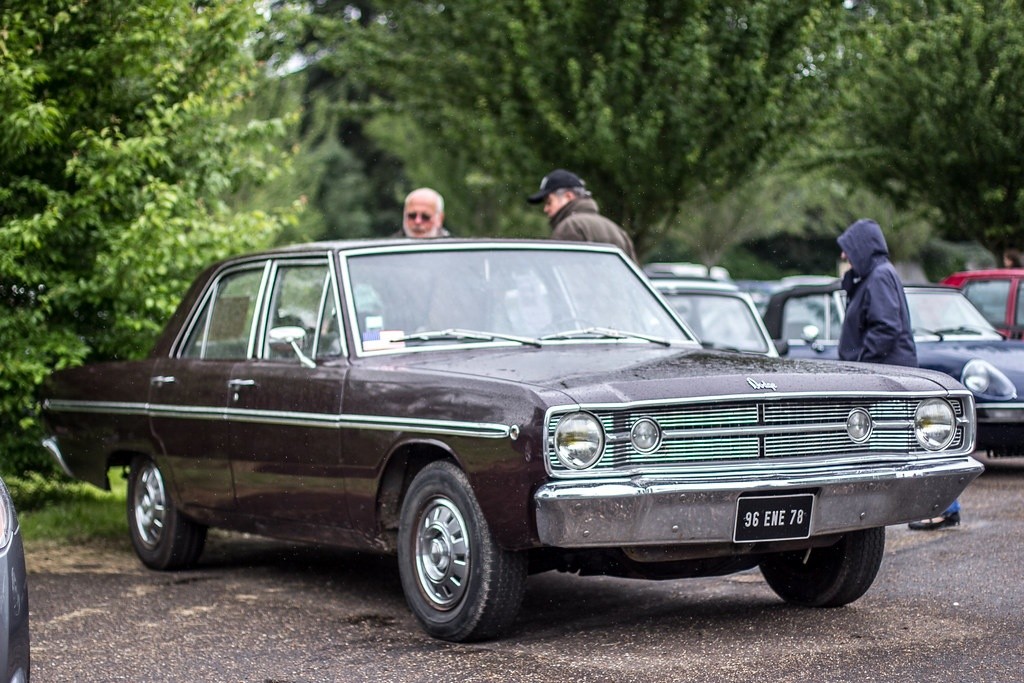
[909,512,961,530]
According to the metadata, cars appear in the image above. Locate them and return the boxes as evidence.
[30,237,986,641]
[636,265,1024,465]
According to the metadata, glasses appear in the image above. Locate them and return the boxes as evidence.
[406,211,434,222]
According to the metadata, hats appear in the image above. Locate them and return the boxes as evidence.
[526,168,584,206]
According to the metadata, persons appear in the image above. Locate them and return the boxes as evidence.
[369,188,490,337]
[837,218,960,530]
[1003,251,1023,268]
[527,170,642,332]
